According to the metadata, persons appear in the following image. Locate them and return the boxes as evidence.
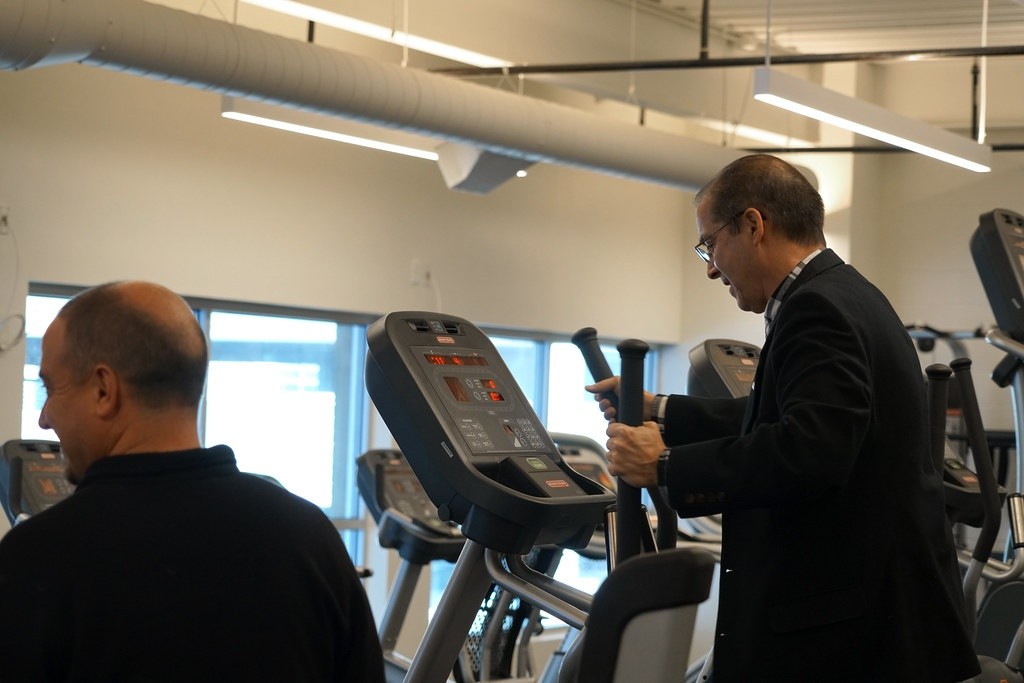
[0,280,387,683]
[586,155,982,683]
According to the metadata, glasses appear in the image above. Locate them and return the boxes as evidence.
[694,212,765,264]
